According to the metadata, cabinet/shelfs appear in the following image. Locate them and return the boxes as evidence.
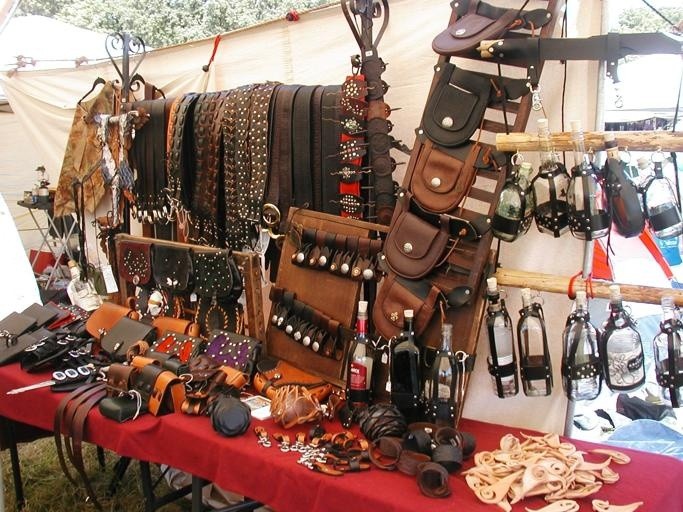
[0,360,683,512]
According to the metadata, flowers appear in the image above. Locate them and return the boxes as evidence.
[35,165,51,187]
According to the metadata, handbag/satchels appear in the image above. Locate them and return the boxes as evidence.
[152,246,194,296]
[86,298,139,342]
[135,364,187,416]
[126,341,161,368]
[253,357,332,403]
[218,365,250,389]
[100,390,148,423]
[205,330,262,375]
[101,316,158,362]
[151,316,200,341]
[135,286,185,324]
[119,241,155,288]
[148,351,191,376]
[106,362,138,397]
[194,250,243,303]
[195,298,244,340]
[147,330,206,364]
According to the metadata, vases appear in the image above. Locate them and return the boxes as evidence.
[38,186,50,203]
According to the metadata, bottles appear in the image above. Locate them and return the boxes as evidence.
[32,184,39,205]
[390,309,425,418]
[347,301,376,409]
[532,118,572,238]
[637,159,683,240]
[517,288,553,396]
[603,133,645,238]
[67,260,103,311]
[39,179,49,205]
[561,291,602,400]
[601,284,646,394]
[653,296,683,388]
[430,324,459,418]
[24,191,32,204]
[484,277,519,399]
[492,162,536,243]
[567,120,612,240]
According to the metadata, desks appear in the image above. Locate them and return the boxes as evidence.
[15,200,85,291]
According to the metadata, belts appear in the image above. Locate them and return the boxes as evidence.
[311,85,324,211]
[73,389,107,510]
[235,86,251,247]
[127,100,172,237]
[54,381,103,489]
[166,89,234,246]
[263,91,286,226]
[262,85,284,193]
[246,82,279,223]
[65,384,106,463]
[267,84,304,238]
[293,85,319,210]
[322,85,341,216]
